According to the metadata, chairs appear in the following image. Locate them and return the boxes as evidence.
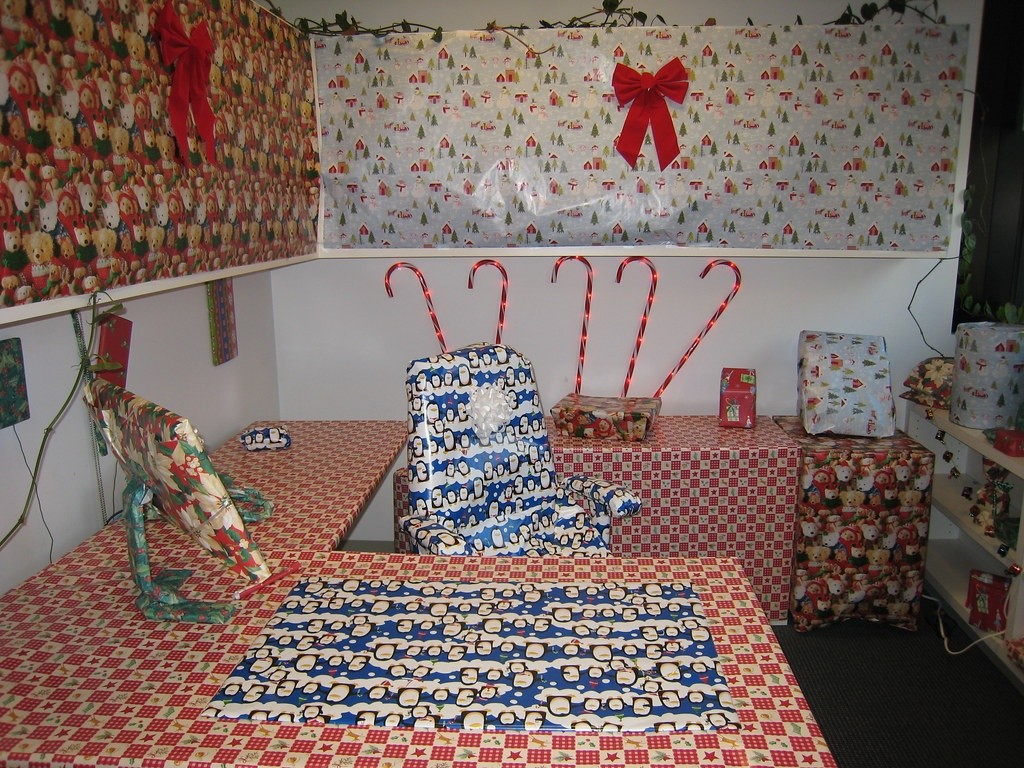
[398,343,642,557]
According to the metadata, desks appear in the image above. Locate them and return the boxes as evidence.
[773,415,937,628]
[0,417,841,768]
[390,414,801,624]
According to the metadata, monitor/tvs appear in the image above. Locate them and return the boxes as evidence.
[84,377,271,624]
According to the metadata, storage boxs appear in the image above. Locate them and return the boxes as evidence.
[796,329,896,437]
[719,368,756,427]
[993,429,1024,457]
[548,393,663,442]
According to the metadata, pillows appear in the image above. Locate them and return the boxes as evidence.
[899,356,954,410]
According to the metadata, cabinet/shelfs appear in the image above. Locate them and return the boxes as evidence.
[903,401,1024,696]
